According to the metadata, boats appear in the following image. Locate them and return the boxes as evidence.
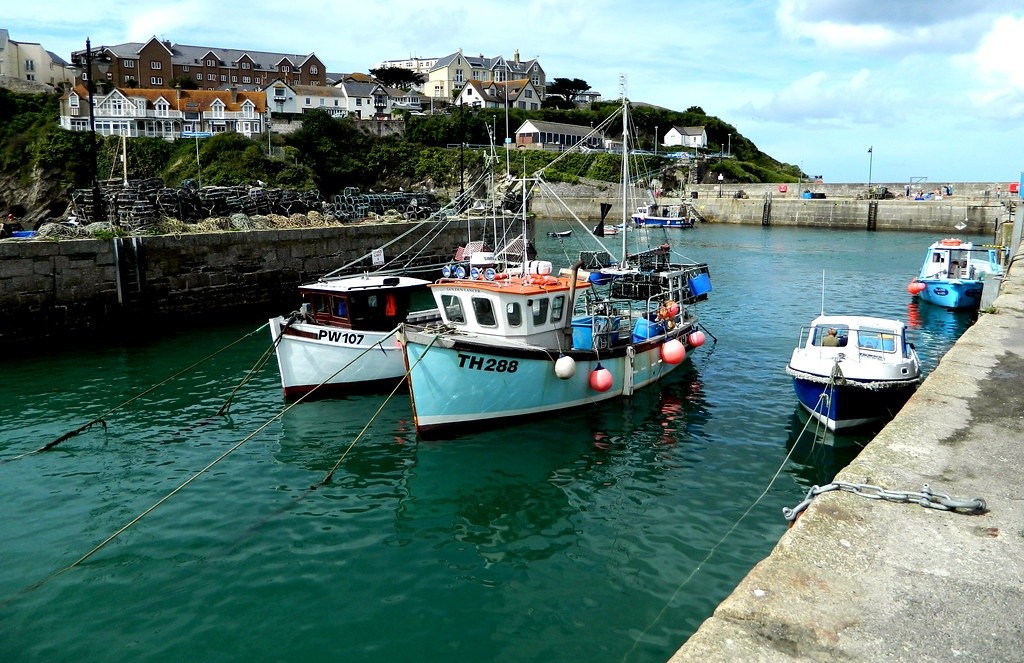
[612,243,711,304]
[615,223,633,231]
[546,229,573,237]
[396,66,705,433]
[908,236,984,307]
[267,112,537,400]
[785,314,926,431]
[593,224,619,236]
[630,203,696,228]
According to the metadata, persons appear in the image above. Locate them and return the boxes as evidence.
[996,188,1000,199]
[822,327,840,347]
[905,183,952,200]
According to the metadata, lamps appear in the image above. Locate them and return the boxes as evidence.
[442,265,497,280]
